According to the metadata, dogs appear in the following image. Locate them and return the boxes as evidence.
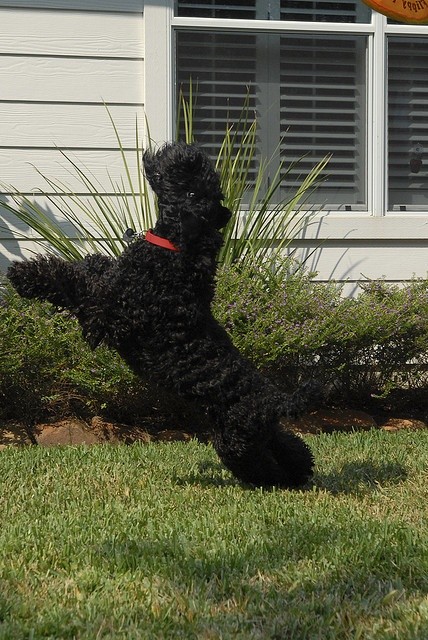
[6,141,314,491]
